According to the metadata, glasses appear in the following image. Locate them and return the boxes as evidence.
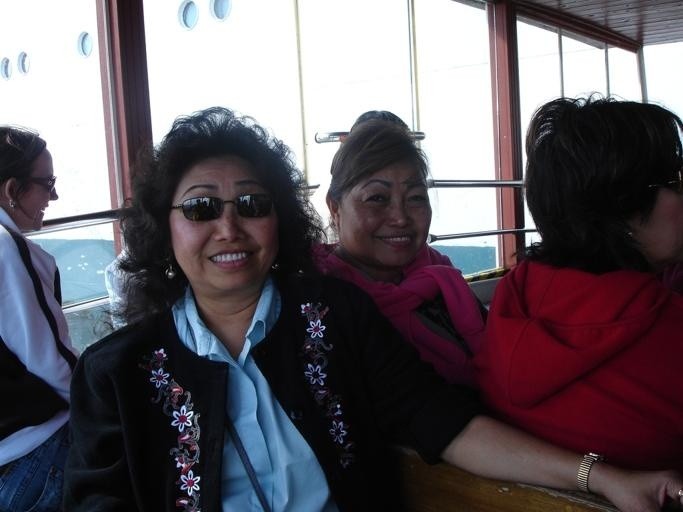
[169,192,274,221]
[649,172,682,188]
[20,174,57,192]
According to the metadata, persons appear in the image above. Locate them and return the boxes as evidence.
[317,110,493,416]
[58,107,683,512]
[473,88,683,468]
[1,125,80,512]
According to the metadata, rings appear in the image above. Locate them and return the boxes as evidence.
[676,487,683,498]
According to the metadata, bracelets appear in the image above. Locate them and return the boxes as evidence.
[576,450,612,496]
